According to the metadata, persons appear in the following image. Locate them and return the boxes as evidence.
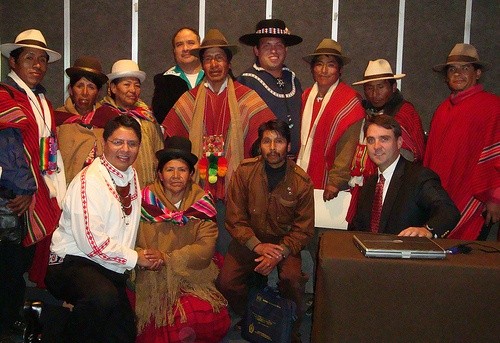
[0,29,67,343]
[346,112,461,238]
[234,19,303,159]
[219,118,314,343]
[127,134,232,343]
[431,42,500,241]
[353,58,425,177]
[54,54,114,188]
[151,28,208,123]
[106,58,165,187]
[165,28,276,330]
[44,113,162,343]
[295,37,365,262]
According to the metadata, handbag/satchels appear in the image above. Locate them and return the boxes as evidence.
[0,197,28,247]
[241,288,297,343]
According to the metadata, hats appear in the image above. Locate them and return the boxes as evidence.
[301,39,353,67]
[155,136,199,166]
[65,55,109,85]
[432,44,490,72]
[239,19,303,47]
[105,60,146,85]
[189,29,240,58]
[352,59,406,86]
[0,29,62,63]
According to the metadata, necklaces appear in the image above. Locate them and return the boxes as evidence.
[30,95,53,135]
[112,182,133,216]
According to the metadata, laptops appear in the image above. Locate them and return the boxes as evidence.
[353,234,446,260]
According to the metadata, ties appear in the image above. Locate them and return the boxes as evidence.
[368,174,386,233]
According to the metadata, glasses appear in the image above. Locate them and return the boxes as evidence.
[106,139,140,149]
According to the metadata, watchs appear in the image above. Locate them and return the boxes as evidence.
[425,225,435,236]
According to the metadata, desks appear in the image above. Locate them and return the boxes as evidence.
[309,229,500,343]
